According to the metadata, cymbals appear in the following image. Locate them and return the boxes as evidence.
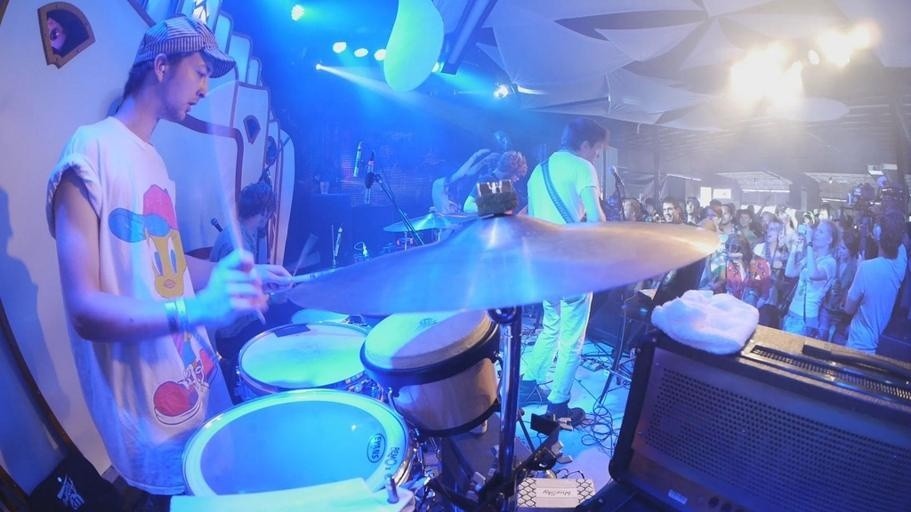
[287,213,724,316]
[383,213,475,232]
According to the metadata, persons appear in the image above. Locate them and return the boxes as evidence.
[463,149,529,219]
[432,146,502,212]
[590,178,907,383]
[518,115,610,428]
[208,179,278,353]
[44,12,299,509]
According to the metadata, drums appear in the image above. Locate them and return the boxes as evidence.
[359,310,500,433]
[234,322,386,404]
[291,307,352,325]
[181,390,436,511]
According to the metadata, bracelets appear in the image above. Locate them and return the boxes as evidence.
[160,296,179,334]
[173,294,189,334]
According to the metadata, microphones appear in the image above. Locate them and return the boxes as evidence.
[353,143,362,178]
[608,165,625,188]
[364,153,374,204]
[334,227,343,257]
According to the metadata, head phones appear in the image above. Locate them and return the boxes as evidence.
[872,209,905,241]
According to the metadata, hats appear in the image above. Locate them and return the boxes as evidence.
[132,15,235,78]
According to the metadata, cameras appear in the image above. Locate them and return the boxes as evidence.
[797,225,806,234]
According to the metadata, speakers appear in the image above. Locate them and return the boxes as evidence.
[587,298,653,356]
[608,321,911,512]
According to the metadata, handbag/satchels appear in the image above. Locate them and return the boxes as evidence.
[802,326,821,339]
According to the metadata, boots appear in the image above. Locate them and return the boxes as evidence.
[545,400,584,427]
[518,374,550,407]
